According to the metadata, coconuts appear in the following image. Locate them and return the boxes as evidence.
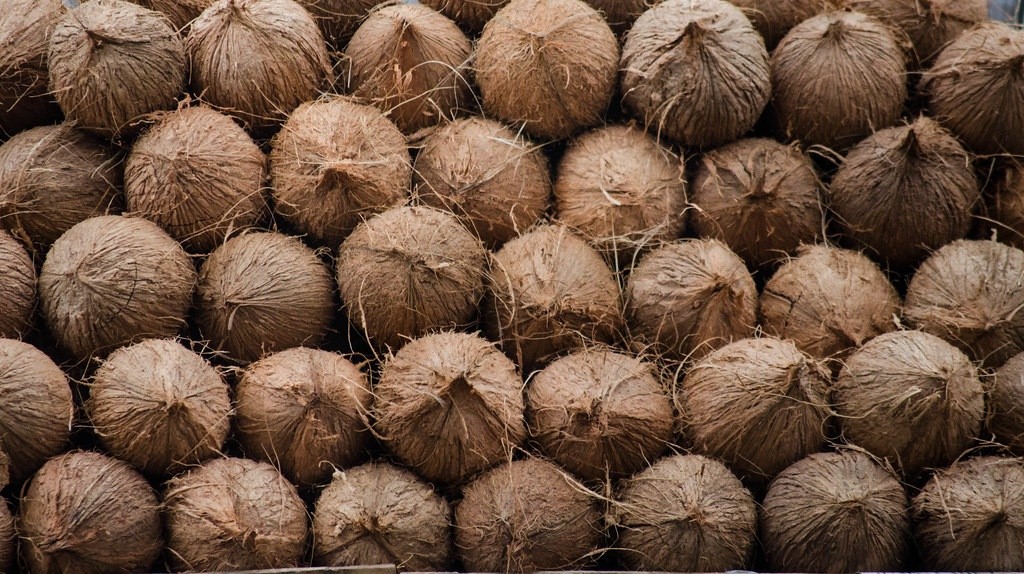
[0,0,1024,574]
